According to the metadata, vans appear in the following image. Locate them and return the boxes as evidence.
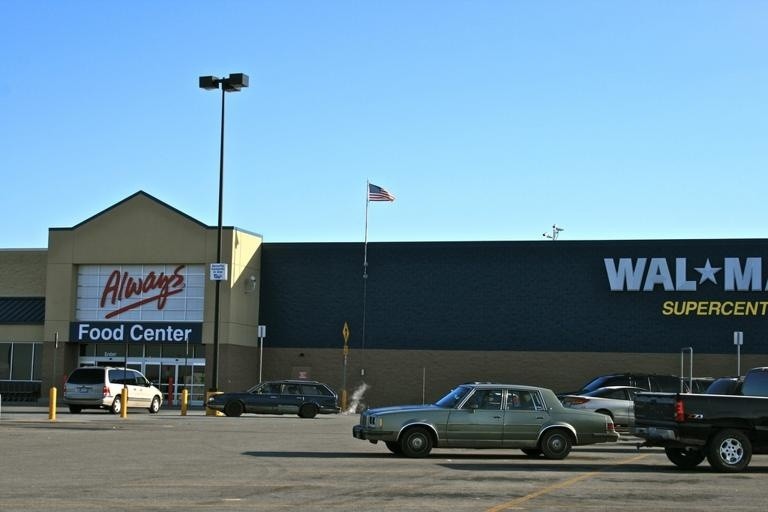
[63,365,163,413]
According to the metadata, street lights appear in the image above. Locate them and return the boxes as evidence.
[200,72,248,387]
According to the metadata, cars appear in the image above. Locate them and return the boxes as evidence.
[558,374,741,431]
[206,379,341,418]
[352,384,619,459]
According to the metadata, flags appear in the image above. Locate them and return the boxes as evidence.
[368,183,395,201]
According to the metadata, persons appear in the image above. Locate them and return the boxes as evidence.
[270,387,277,393]
[511,395,521,409]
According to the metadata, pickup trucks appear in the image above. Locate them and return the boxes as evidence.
[634,367,767,473]
